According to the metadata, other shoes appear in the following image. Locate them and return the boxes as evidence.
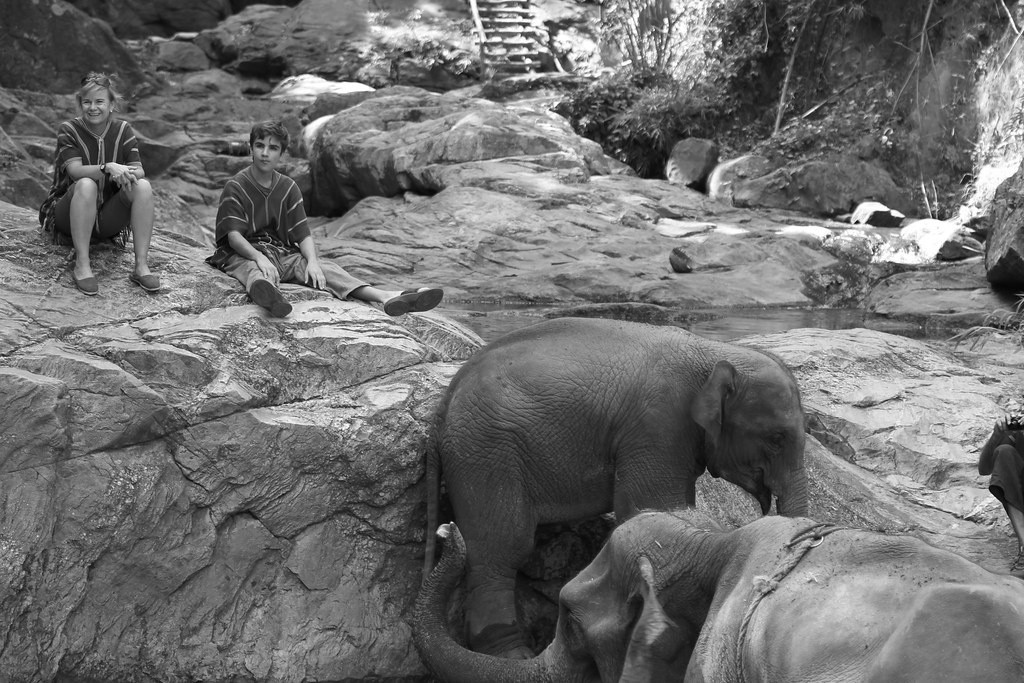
[71,271,98,295]
[1010,552,1024,578]
[129,274,160,291]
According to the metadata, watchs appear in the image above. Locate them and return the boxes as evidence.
[100,163,106,175]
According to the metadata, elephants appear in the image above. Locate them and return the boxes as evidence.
[414,509,1024,683]
[423,317,809,657]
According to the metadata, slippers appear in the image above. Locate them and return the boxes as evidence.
[249,278,293,318]
[384,288,444,317]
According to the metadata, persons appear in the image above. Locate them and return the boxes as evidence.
[39,72,161,295]
[204,122,444,319]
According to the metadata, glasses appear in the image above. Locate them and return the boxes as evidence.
[80,77,110,87]
